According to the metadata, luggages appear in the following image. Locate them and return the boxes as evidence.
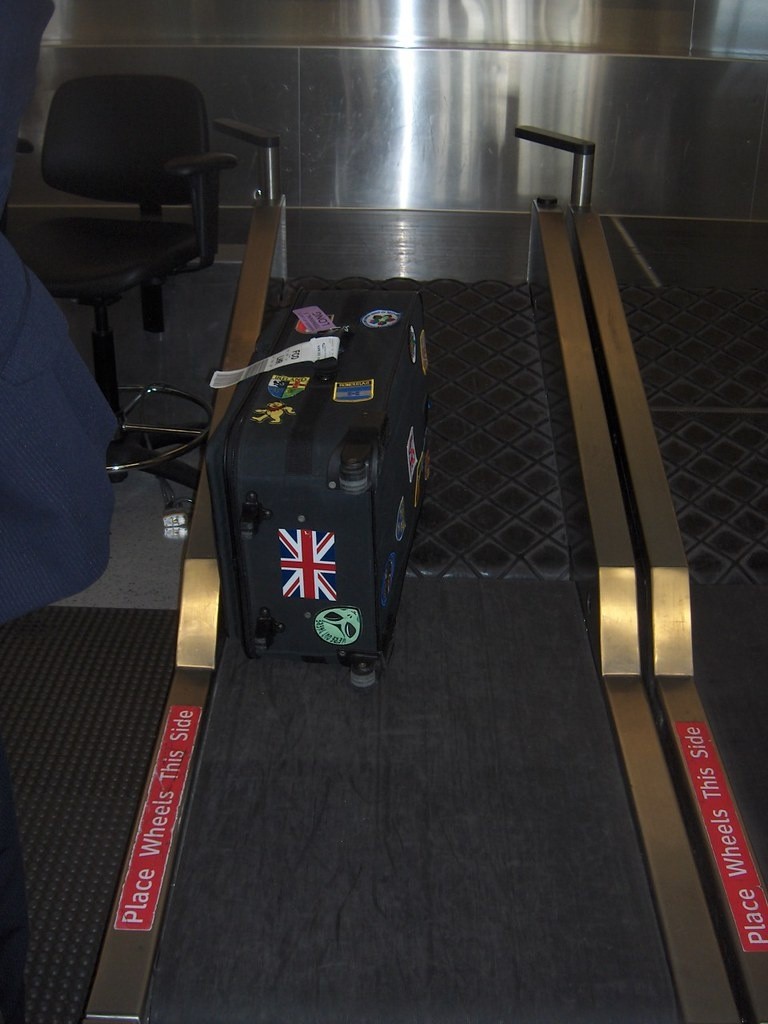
[205,280,428,692]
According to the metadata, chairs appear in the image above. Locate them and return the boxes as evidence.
[0,75,238,483]
